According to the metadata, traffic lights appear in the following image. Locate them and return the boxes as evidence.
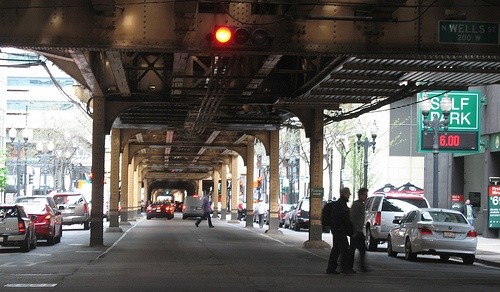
[208,25,275,52]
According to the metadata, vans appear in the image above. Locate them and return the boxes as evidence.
[182,195,207,220]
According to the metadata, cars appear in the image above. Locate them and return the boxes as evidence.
[52,187,120,229]
[0,204,38,253]
[210,202,222,213]
[145,200,183,219]
[237,203,297,229]
[13,194,66,246]
[387,208,479,266]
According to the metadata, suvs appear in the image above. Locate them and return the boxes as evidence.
[290,196,330,233]
[364,191,431,252]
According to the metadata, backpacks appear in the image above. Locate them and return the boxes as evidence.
[321,200,337,228]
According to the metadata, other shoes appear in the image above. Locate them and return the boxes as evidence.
[209,226,215,228]
[340,268,356,274]
[326,270,340,275]
[195,223,199,227]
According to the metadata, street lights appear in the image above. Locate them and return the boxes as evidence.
[8,124,30,195]
[265,158,269,203]
[285,152,300,204]
[56,151,71,192]
[353,119,378,189]
[227,182,232,212]
[419,92,452,219]
[36,141,54,194]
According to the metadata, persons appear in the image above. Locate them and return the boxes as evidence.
[461,199,477,226]
[195,191,214,228]
[238,201,244,221]
[326,187,357,275]
[348,188,372,273]
[253,199,265,229]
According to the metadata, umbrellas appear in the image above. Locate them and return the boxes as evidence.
[373,182,424,195]
